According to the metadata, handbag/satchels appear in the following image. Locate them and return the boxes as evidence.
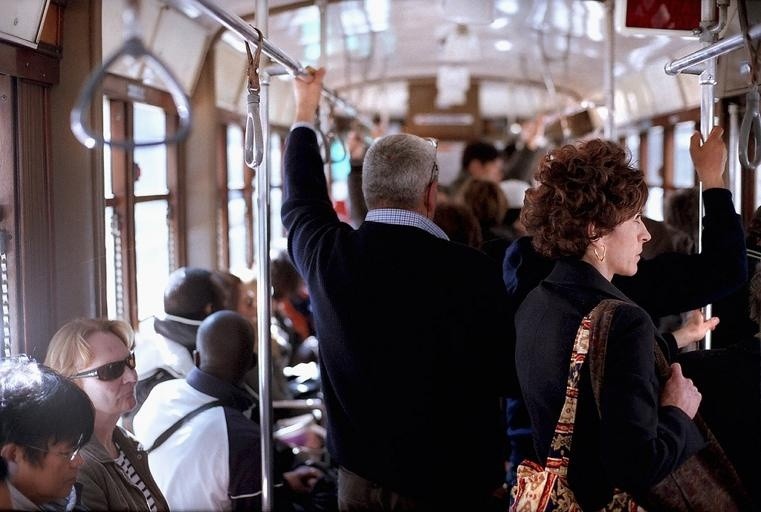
[511,298,741,511]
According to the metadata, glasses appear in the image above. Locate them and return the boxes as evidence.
[66,353,137,384]
[15,440,81,463]
[424,137,439,186]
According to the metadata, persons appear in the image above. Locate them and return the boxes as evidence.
[280,63,508,509]
